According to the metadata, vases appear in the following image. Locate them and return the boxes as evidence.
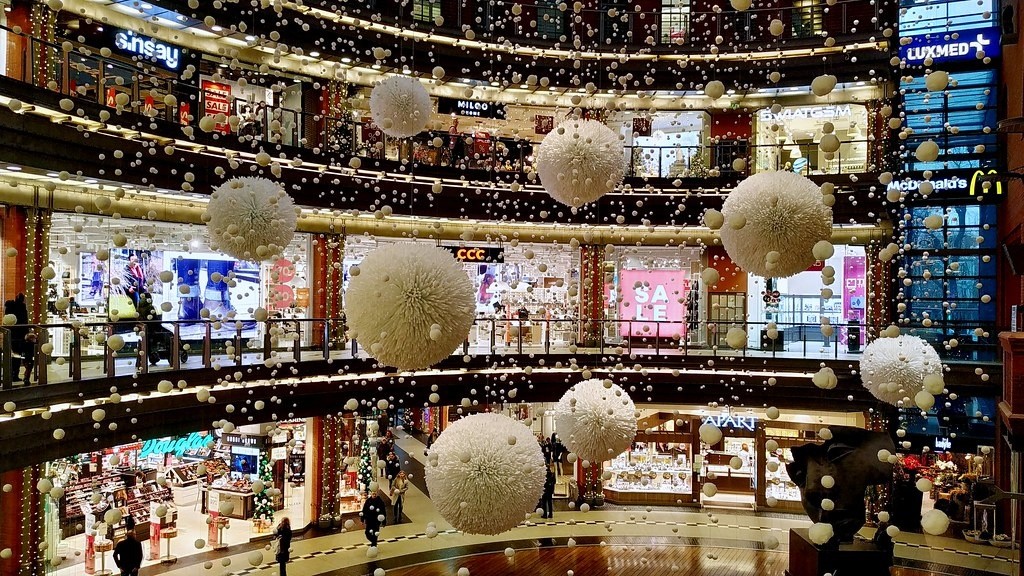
[989,539,1012,548]
[962,529,989,543]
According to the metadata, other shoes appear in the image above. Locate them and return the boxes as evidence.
[541,515,547,519]
[547,515,553,518]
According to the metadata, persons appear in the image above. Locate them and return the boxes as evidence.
[173,258,257,331]
[275,517,292,576]
[113,530,142,576]
[377,430,409,522]
[361,491,386,547]
[5,292,37,386]
[540,432,563,519]
[426,428,437,449]
[448,119,459,167]
[122,254,144,308]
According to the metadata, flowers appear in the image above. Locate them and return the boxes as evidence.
[965,528,981,536]
[996,533,1011,541]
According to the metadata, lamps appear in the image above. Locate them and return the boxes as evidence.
[847,114,861,137]
[778,118,801,158]
[812,126,825,143]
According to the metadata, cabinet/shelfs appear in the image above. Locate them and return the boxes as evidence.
[114,480,178,548]
[60,473,127,539]
[778,295,842,344]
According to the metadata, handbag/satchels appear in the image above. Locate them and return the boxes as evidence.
[269,537,281,556]
[389,488,394,496]
